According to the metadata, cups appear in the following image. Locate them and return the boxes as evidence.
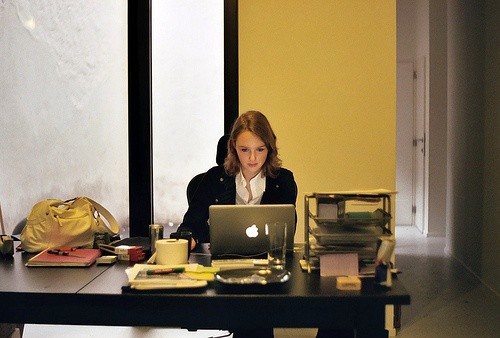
[149,224,164,253]
[264,222,288,269]
[171,231,192,260]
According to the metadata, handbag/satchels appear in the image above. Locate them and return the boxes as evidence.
[20,196,120,253]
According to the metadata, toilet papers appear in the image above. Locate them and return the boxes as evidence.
[155,238,189,265]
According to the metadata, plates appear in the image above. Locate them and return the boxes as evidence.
[213,269,291,288]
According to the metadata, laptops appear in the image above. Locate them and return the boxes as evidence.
[194,203,295,264]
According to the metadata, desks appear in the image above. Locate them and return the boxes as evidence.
[0,239,412,338]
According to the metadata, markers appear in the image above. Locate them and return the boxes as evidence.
[147,267,185,275]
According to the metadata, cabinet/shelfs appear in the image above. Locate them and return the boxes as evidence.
[303,193,391,277]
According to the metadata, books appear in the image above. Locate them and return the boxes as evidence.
[24,247,101,267]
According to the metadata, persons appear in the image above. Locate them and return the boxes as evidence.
[170,111,298,250]
